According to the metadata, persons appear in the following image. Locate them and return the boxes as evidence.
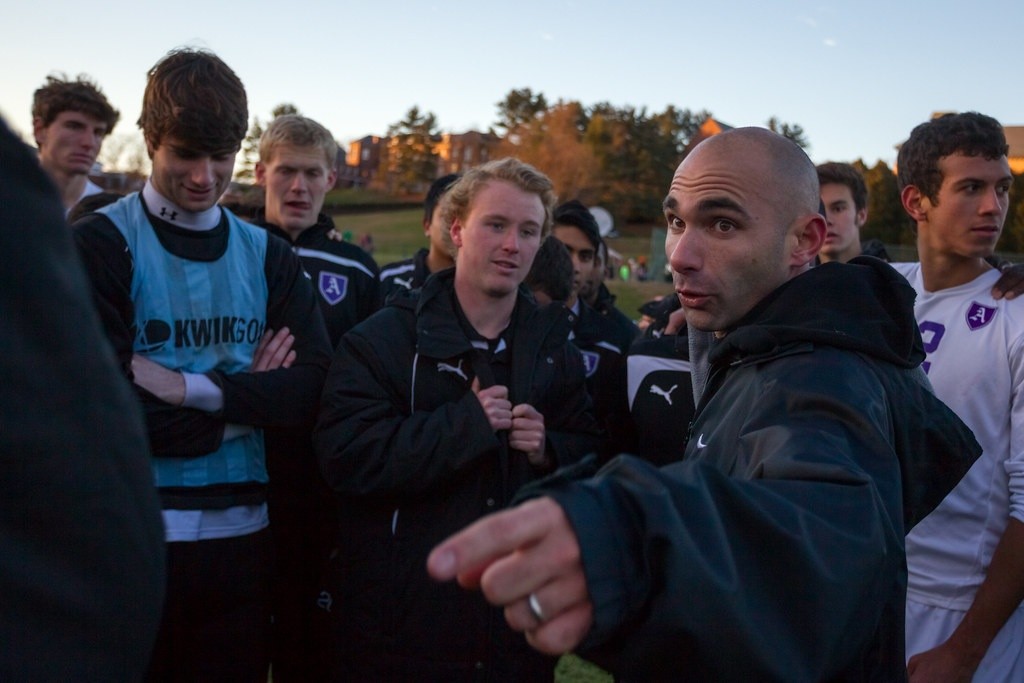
[363,174,461,306]
[248,115,378,350]
[428,125,983,683]
[0,117,166,683]
[32,79,125,221]
[73,51,330,683]
[315,156,603,683]
[885,112,1024,683]
[524,198,1024,466]
[813,161,894,266]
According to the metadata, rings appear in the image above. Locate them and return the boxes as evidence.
[529,593,547,623]
